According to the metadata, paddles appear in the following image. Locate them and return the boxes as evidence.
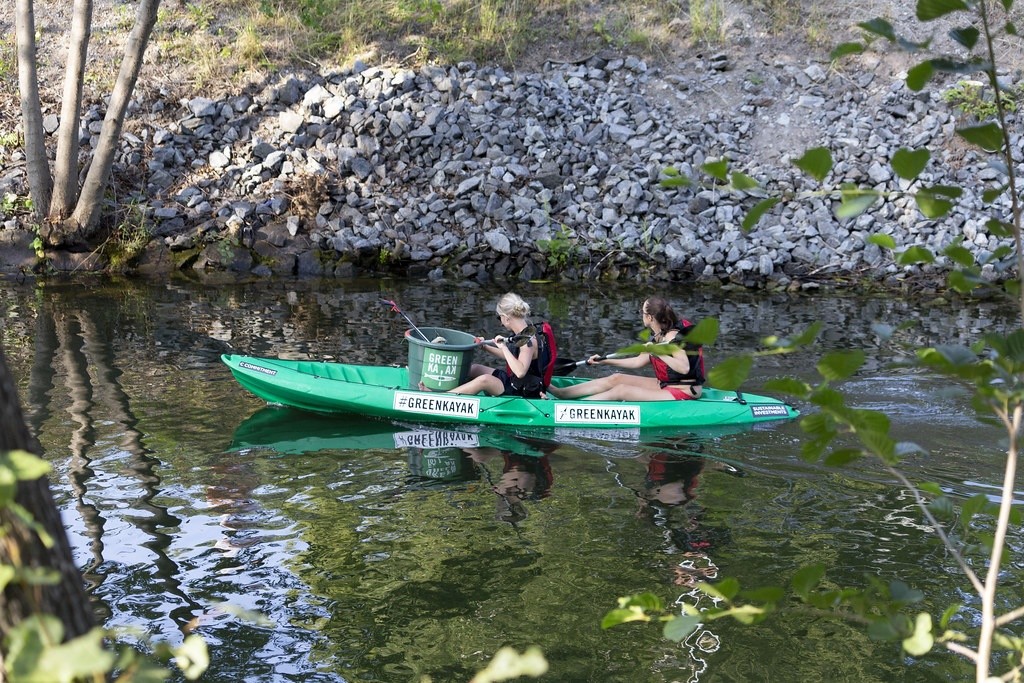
[477,328,537,347]
[549,324,706,376]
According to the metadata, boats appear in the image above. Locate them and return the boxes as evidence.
[221,352,802,440]
[223,401,548,455]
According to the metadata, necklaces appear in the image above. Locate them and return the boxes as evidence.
[653,331,662,337]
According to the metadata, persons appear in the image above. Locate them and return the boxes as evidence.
[418,292,557,400]
[540,295,705,400]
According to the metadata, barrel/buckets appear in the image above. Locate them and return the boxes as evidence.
[404,327,483,393]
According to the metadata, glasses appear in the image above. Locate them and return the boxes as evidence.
[497,315,505,321]
[638,310,652,316]
